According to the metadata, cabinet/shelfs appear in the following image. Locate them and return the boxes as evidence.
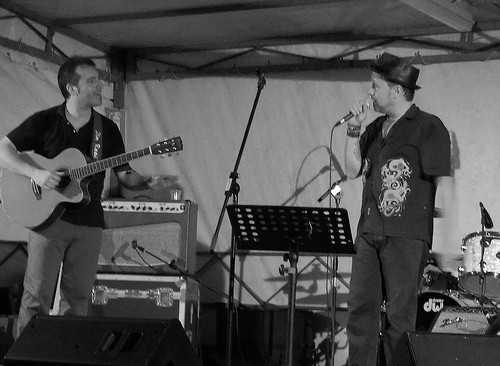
[53,197,199,342]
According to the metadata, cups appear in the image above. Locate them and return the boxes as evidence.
[157,287,174,307]
[92,285,109,305]
[170,189,182,200]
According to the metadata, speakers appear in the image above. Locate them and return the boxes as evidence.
[405,305,500,366]
[3,313,202,366]
[91,198,198,275]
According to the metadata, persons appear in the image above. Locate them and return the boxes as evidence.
[0,55,180,339]
[343,52,456,366]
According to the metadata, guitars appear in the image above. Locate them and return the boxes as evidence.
[0,136,184,233]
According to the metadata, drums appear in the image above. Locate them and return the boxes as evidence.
[416,266,497,332]
[457,231,500,300]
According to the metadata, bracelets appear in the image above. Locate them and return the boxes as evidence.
[347,124,361,137]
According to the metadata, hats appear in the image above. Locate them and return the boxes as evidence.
[367,52,422,90]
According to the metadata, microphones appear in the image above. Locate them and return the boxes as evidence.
[479,202,494,229]
[336,101,371,126]
[131,238,137,249]
[317,178,345,202]
[255,68,267,85]
